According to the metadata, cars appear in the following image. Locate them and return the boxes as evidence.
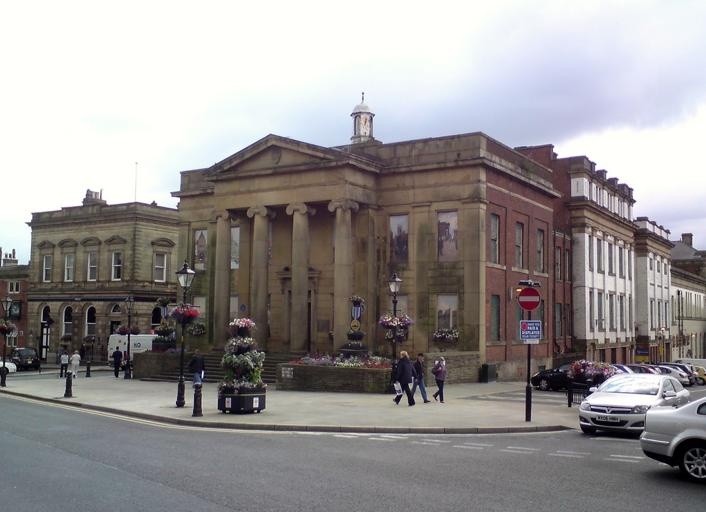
[639,396,705,486]
[578,372,691,437]
[0,360,17,376]
[607,358,705,387]
[531,363,605,394]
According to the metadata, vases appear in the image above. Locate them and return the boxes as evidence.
[217,387,266,414]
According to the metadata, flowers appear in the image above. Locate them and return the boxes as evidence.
[170,305,199,325]
[0,318,15,336]
[217,315,267,388]
[379,313,412,330]
[114,324,140,335]
[570,358,620,383]
[432,325,461,345]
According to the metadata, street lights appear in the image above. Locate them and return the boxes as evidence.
[517,279,541,423]
[658,328,667,362]
[386,270,405,380]
[0,294,14,388]
[123,294,134,380]
[172,258,197,408]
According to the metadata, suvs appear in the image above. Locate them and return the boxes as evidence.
[8,347,40,372]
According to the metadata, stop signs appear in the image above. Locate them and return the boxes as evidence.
[517,287,542,312]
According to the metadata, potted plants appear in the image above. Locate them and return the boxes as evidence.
[348,294,364,308]
[151,321,176,354]
[344,328,366,350]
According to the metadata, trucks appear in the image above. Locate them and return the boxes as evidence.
[105,333,162,370]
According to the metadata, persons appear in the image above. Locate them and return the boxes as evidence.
[411,353,431,403]
[60,351,68,378]
[69,348,81,379]
[393,351,417,407]
[112,346,122,377]
[185,348,205,387]
[432,356,448,403]
